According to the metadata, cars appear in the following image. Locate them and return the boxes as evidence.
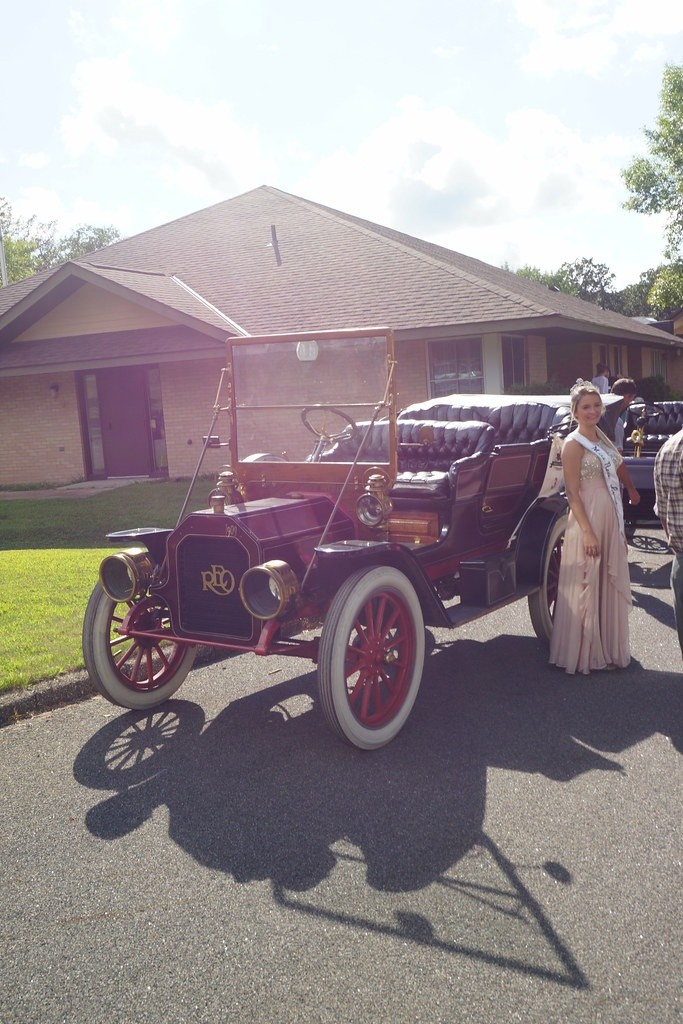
[82,325,625,755]
[620,401,682,537]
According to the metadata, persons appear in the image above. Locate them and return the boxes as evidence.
[652,428,683,660]
[549,378,641,675]
[592,364,609,394]
[610,377,642,506]
